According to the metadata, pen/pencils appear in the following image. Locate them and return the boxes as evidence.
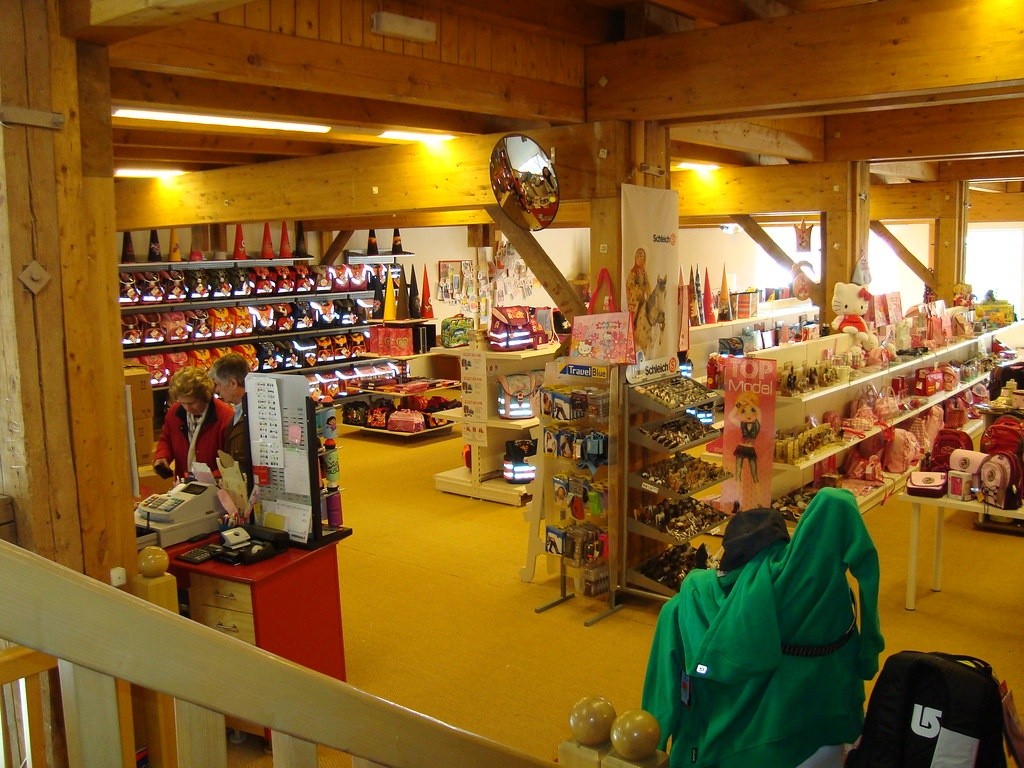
[218,507,245,526]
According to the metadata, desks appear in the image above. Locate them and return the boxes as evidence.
[896,492,1024,611]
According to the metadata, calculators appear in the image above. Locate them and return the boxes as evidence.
[175,542,223,564]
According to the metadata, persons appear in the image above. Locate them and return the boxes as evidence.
[207,353,252,463]
[560,435,572,458]
[555,486,566,504]
[548,534,561,554]
[546,432,556,453]
[152,366,235,486]
[543,393,553,415]
[542,167,557,193]
[555,399,569,420]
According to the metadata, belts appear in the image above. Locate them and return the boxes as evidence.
[781,585,857,658]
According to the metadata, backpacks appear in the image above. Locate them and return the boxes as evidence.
[845,649,1007,768]
[845,382,1024,511]
[489,306,571,352]
[496,369,545,420]
[504,439,537,484]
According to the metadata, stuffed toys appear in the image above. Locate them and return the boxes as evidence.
[830,281,872,341]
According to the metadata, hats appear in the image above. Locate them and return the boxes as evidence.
[719,508,791,571]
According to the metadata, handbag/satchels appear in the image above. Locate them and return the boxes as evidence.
[441,314,474,348]
[569,267,636,367]
[342,395,461,433]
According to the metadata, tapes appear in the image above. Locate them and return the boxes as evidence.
[251,545,263,553]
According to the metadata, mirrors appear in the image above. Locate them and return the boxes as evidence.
[489,135,558,232]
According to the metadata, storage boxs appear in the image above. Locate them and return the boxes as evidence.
[123,363,177,498]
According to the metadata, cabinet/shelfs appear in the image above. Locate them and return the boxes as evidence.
[685,307,1021,527]
[617,373,735,602]
[521,353,623,627]
[430,330,566,504]
[166,520,350,753]
[114,250,426,405]
